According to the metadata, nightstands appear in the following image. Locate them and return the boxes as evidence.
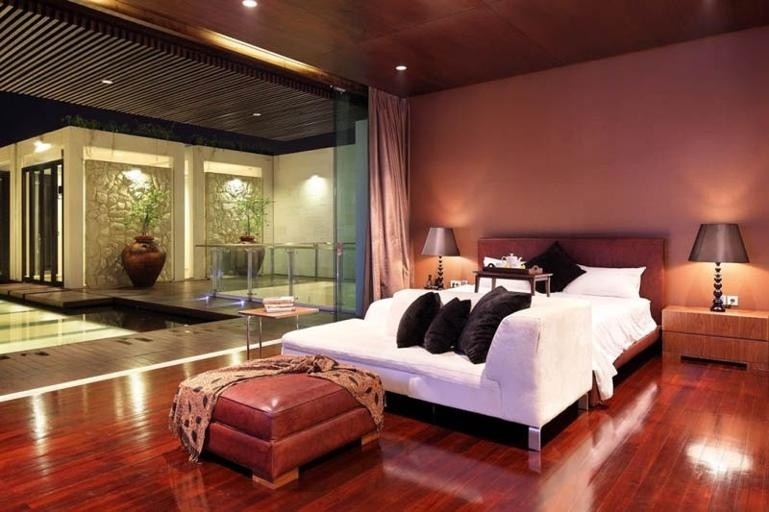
[660,303,769,373]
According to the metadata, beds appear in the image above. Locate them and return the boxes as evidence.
[363,236,668,412]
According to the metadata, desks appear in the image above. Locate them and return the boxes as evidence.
[236,306,320,360]
[472,269,553,297]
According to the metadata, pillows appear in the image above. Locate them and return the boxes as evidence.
[564,263,647,300]
[424,297,473,353]
[526,241,587,293]
[456,284,533,364]
[480,255,531,290]
[396,289,441,350]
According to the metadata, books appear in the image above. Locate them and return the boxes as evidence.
[266,307,296,312]
[264,303,294,308]
[262,296,294,304]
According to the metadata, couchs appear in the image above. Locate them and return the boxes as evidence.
[279,289,592,453]
[180,353,384,490]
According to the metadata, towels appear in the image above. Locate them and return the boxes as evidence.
[262,295,298,312]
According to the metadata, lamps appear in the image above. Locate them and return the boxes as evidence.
[422,226,461,288]
[688,222,749,312]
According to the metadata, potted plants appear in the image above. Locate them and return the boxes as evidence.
[227,190,275,276]
[121,186,168,290]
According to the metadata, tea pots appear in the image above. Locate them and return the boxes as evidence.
[501,253,523,266]
